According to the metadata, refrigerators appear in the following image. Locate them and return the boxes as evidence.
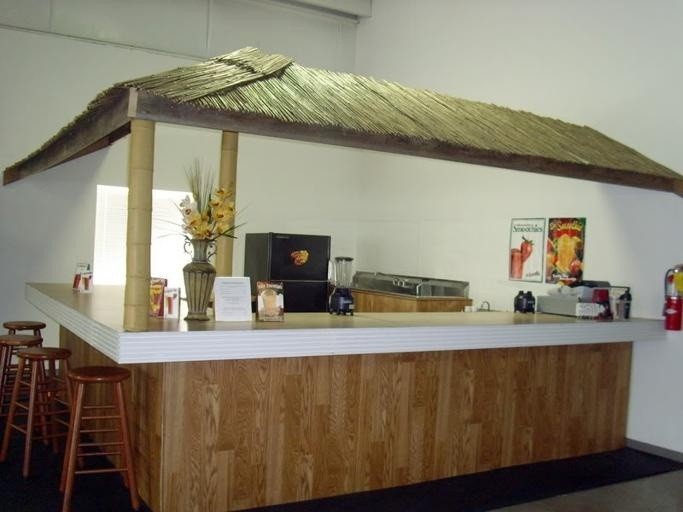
[244,231,331,314]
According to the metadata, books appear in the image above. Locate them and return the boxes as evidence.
[162,286,180,321]
[71,263,89,291]
[149,277,167,319]
[79,270,92,293]
[255,280,285,321]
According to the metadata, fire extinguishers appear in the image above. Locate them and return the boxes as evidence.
[662,264,683,330]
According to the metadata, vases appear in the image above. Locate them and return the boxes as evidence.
[183,238,217,323]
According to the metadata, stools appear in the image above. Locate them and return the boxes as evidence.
[0,320,139,510]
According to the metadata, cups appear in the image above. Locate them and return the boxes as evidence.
[664,296,682,331]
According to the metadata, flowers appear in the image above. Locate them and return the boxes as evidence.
[173,158,245,240]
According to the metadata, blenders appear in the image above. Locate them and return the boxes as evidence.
[330,257,357,318]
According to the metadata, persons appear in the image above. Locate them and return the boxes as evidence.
[544,232,559,282]
[571,242,582,277]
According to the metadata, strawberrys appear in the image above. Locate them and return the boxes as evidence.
[520,240,533,261]
